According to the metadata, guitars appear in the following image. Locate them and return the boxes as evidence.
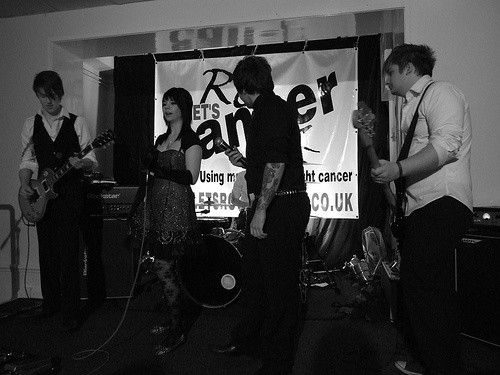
[353,106,404,238]
[17,128,115,222]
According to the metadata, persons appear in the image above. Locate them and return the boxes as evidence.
[18,71,99,333]
[207,56,311,375]
[370,44,473,375]
[126,88,202,334]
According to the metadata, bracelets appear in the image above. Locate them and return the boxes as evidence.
[396,161,403,178]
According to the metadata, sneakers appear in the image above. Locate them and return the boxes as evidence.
[395,361,426,375]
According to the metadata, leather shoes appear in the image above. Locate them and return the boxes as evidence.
[256,364,293,375]
[207,339,245,357]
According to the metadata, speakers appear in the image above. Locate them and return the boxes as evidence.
[454,235,499,346]
[74,217,137,300]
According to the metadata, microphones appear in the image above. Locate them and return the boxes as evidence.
[215,136,248,168]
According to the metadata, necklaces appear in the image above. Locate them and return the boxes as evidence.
[167,132,177,150]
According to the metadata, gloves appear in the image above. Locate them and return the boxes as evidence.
[139,157,193,186]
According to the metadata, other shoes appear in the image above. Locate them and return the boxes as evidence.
[62,313,82,332]
[150,323,189,357]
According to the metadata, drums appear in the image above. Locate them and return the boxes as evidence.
[176,228,253,311]
